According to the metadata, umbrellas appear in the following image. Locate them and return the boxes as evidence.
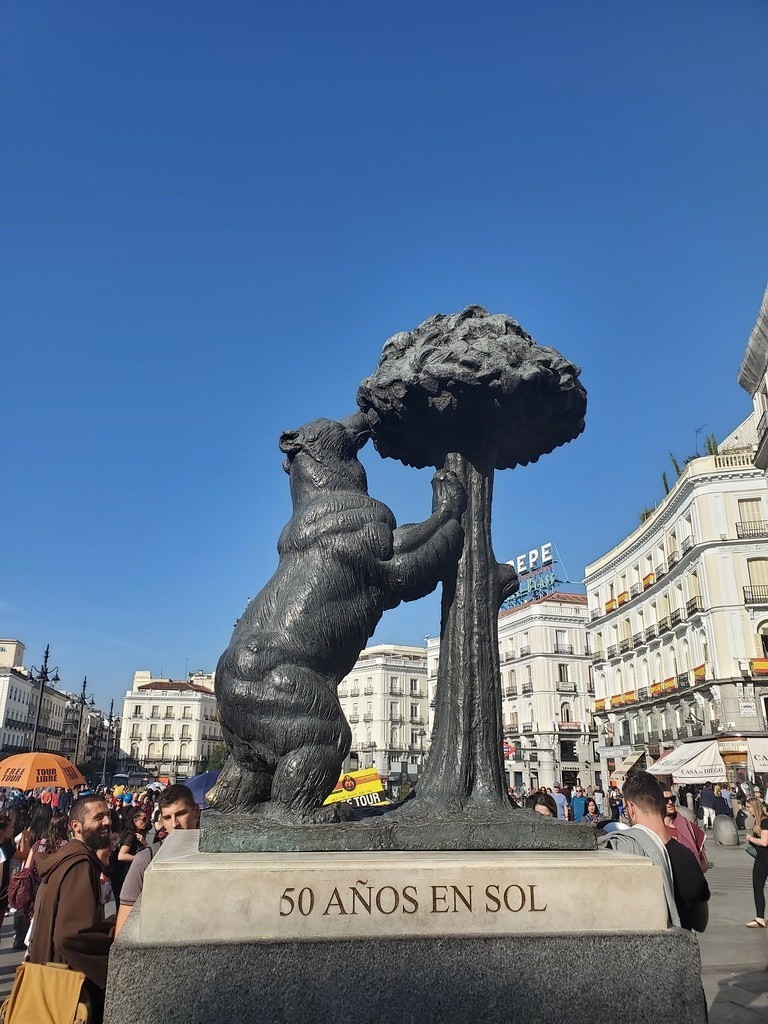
[182,770,219,805]
[0,752,88,792]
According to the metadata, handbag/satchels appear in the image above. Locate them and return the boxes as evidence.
[0,961,88,1024]
[610,797,617,806]
[746,840,757,859]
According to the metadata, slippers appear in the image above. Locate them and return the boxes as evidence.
[746,920,767,928]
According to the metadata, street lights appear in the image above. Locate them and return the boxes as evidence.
[100,700,120,786]
[70,676,95,767]
[24,643,60,753]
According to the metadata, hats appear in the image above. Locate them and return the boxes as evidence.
[553,782,560,787]
[117,794,123,799]
[123,793,133,803]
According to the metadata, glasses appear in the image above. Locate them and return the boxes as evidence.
[138,817,147,822]
[664,795,676,804]
[622,802,634,819]
[576,790,582,792]
[117,800,122,802]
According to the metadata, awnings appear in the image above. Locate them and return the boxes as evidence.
[747,737,768,785]
[611,751,644,779]
[644,740,728,783]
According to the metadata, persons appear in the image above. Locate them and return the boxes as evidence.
[384,790,394,803]
[508,772,767,933]
[0,784,201,1024]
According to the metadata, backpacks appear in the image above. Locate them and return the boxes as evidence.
[8,867,38,915]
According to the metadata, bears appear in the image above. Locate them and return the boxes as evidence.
[202,414,466,826]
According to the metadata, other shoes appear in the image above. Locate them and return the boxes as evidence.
[13,942,27,949]
